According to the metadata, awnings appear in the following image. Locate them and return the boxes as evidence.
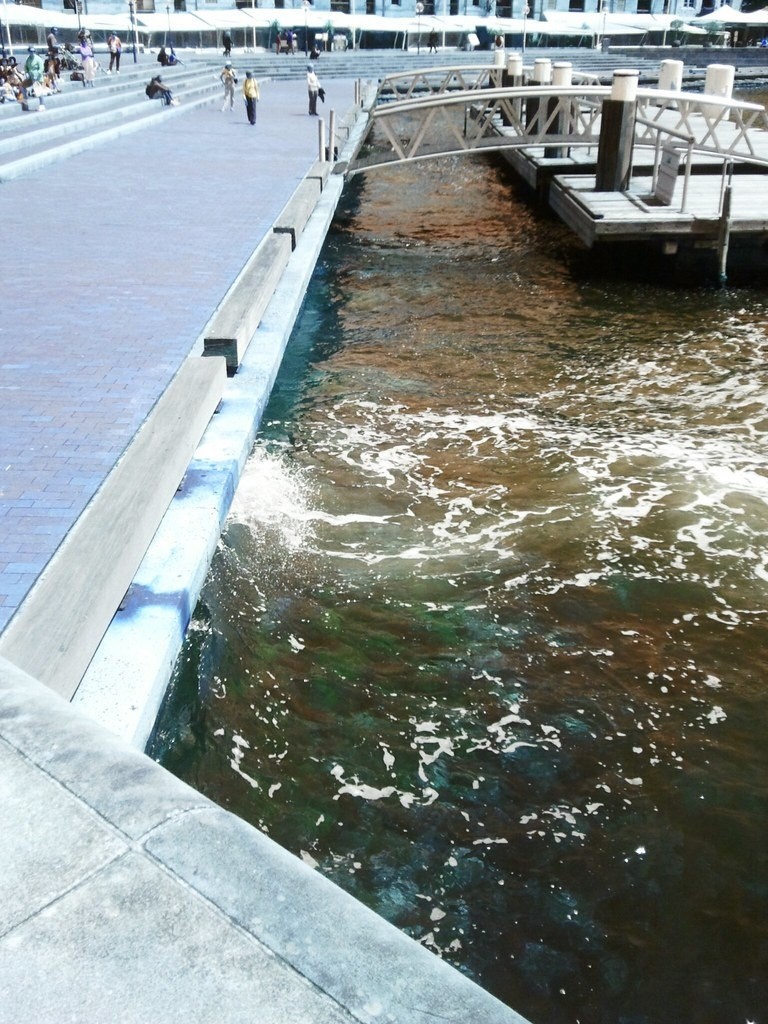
[0,4,708,50]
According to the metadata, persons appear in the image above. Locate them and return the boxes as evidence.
[223,31,233,57]
[78,27,93,53]
[81,38,95,86]
[22,46,61,111]
[286,29,295,55]
[0,56,24,103]
[47,27,61,78]
[243,70,258,125]
[148,76,180,106]
[157,45,184,65]
[220,61,236,112]
[276,31,281,54]
[307,65,320,115]
[429,28,438,53]
[310,43,320,59]
[496,35,504,48]
[107,31,121,74]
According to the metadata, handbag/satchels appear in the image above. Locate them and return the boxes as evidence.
[234,79,238,84]
[70,72,84,81]
[427,42,431,46]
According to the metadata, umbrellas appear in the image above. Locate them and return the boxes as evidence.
[744,7,768,40]
[690,4,744,41]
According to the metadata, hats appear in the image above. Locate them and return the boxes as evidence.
[225,60,231,66]
[246,72,252,78]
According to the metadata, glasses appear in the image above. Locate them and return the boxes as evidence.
[29,51,36,53]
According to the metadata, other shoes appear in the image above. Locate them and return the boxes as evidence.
[170,100,179,105]
[107,70,111,74]
[252,122,255,124]
[310,113,318,115]
[117,71,120,74]
[38,105,45,111]
